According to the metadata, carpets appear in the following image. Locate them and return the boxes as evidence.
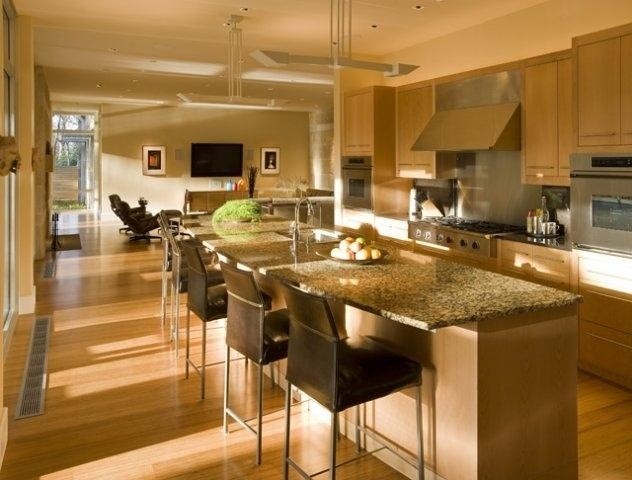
[46,233,82,252]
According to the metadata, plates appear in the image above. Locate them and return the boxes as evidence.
[314,247,389,264]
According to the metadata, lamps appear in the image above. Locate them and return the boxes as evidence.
[248,0,422,80]
[172,11,292,116]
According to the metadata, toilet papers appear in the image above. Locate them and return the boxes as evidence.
[408,186,418,223]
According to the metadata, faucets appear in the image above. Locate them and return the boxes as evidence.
[293,196,315,239]
[291,243,300,271]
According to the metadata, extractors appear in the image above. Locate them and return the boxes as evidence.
[410,101,521,154]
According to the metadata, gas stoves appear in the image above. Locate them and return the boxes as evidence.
[407,217,526,258]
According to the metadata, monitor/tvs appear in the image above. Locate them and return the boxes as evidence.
[190,142,243,178]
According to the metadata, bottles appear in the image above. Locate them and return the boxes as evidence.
[225,178,246,191]
[525,195,560,234]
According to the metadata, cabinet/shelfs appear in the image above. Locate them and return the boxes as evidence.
[340,86,374,156]
[521,48,573,186]
[396,78,435,179]
[567,246,631,394]
[495,233,571,302]
[339,208,375,234]
[572,23,632,153]
[371,210,408,242]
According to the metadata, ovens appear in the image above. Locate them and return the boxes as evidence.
[341,156,371,211]
[569,152,631,258]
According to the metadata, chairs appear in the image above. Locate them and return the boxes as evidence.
[271,272,428,480]
[216,258,314,468]
[106,191,272,401]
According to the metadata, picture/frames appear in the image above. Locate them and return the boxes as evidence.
[259,146,281,176]
[141,143,168,177]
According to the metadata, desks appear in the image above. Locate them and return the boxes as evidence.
[179,209,583,480]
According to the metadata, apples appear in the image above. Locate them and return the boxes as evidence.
[330,237,382,261]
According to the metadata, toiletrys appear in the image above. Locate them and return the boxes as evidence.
[226,179,241,192]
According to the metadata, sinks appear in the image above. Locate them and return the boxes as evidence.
[275,226,343,244]
